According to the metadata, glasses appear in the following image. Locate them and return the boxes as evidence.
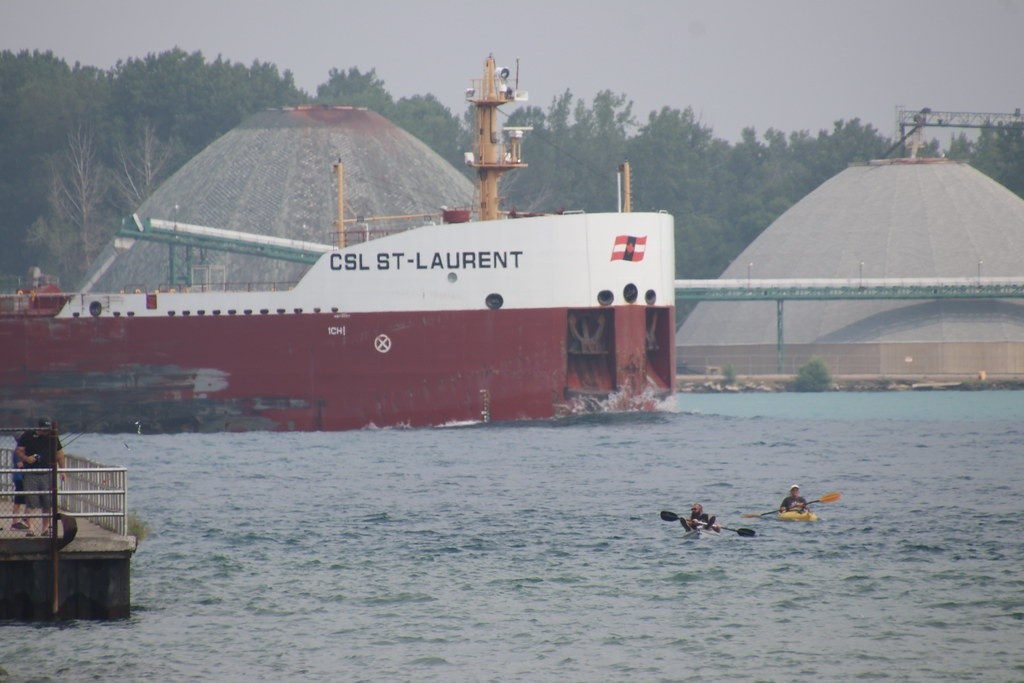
[691,508,698,510]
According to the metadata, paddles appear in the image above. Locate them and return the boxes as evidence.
[741,492,842,519]
[660,510,756,538]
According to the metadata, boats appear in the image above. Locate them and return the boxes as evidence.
[683,527,721,540]
[777,510,817,522]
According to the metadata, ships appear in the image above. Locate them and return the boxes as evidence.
[0,50,678,435]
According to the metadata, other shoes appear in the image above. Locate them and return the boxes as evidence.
[41,530,50,537]
[25,530,35,537]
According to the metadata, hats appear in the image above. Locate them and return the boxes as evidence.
[790,484,799,491]
[38,415,52,431]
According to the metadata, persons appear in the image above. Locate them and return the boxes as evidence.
[780,484,809,514]
[10,415,67,537]
[689,503,708,530]
